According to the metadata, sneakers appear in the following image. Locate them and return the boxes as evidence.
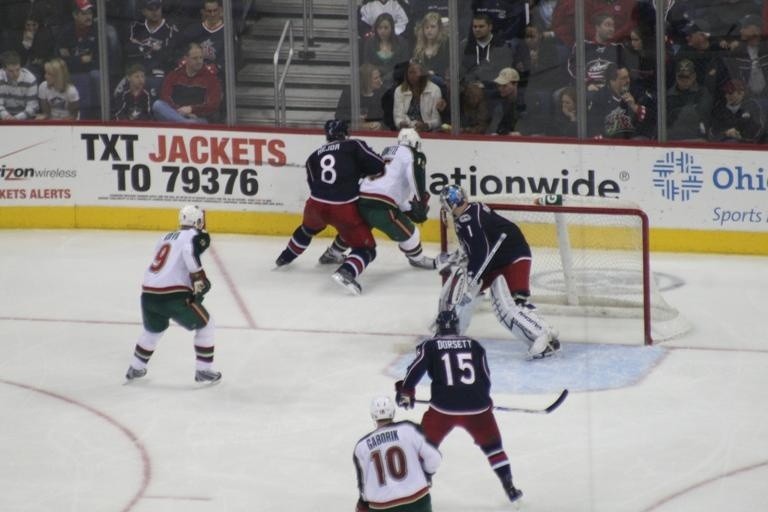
[409,255,436,269]
[277,251,288,265]
[194,370,221,381]
[336,267,362,293]
[126,365,147,379]
[535,339,560,357]
[318,247,346,264]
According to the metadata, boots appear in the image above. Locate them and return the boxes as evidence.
[508,486,522,502]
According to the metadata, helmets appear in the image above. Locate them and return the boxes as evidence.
[437,311,458,334]
[178,204,205,230]
[398,127,421,150]
[324,120,347,140]
[371,396,395,420]
[439,184,467,224]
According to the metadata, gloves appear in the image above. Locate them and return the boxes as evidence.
[191,278,210,296]
[395,381,415,408]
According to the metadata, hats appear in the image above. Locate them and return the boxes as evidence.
[681,18,709,32]
[738,14,761,25]
[492,67,520,85]
[74,0,94,11]
[722,79,743,93]
[142,0,161,9]
[676,59,694,75]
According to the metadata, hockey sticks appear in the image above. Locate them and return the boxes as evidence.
[416,389,568,416]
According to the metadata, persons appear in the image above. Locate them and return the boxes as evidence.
[335,0,768,144]
[353,397,442,512]
[396,312,523,503]
[128,205,222,382]
[434,184,561,352]
[320,127,436,268]
[276,120,385,290]
[0,0,240,124]
